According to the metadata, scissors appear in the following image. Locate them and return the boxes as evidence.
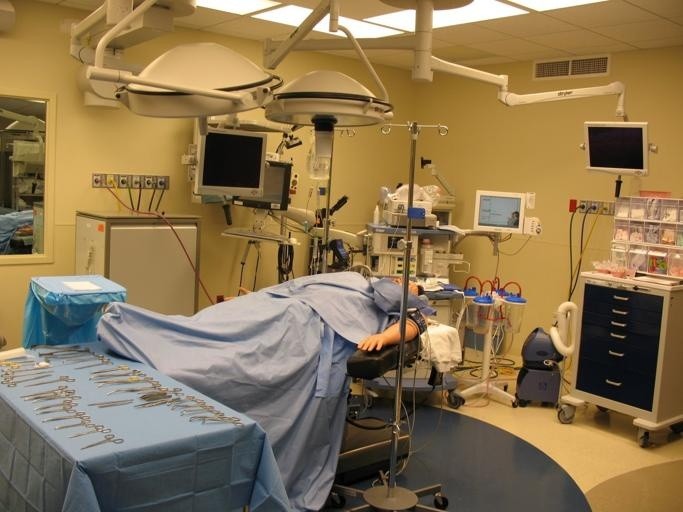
[0,345,244,450]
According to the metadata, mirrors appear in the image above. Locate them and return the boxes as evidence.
[0,90,58,266]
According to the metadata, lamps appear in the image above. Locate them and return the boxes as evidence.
[265,70,391,157]
[127,42,278,136]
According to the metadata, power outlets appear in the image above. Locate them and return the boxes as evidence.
[92,174,170,189]
[579,201,615,215]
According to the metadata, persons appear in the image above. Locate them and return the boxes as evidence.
[356,277,428,352]
[17,224,33,237]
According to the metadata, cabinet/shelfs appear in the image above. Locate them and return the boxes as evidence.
[556,270,683,448]
[75,209,202,317]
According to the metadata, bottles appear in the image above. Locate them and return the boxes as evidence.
[463,288,527,335]
[420,238,434,274]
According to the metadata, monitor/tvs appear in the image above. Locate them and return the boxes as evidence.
[584,121,649,177]
[194,127,267,197]
[232,160,291,210]
[473,190,526,234]
